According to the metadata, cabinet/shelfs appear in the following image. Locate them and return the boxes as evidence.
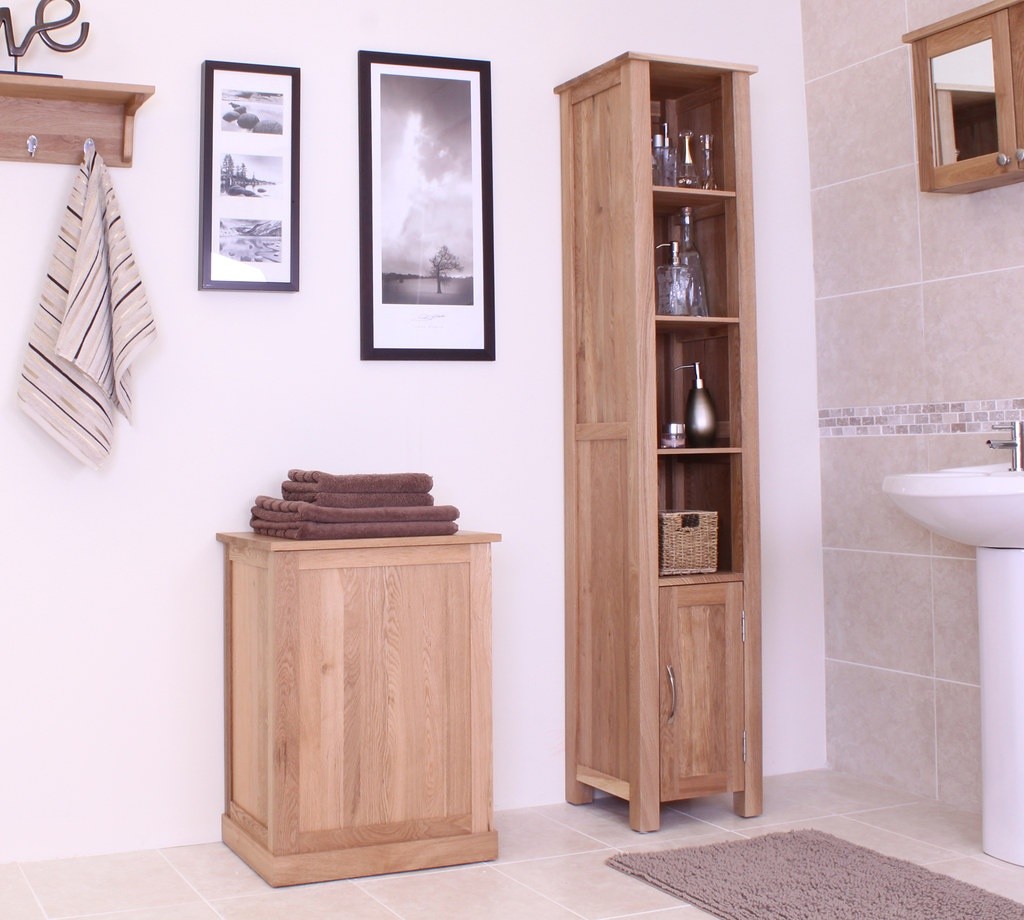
[551,51,764,835]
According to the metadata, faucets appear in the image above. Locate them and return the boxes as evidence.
[986,421,1024,472]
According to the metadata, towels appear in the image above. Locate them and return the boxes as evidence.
[15,146,159,471]
[249,468,461,541]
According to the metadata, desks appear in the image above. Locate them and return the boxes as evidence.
[215,531,503,889]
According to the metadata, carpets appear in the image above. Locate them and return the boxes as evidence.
[605,828,1024,920]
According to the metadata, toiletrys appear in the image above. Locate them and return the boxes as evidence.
[673,362,718,446]
[655,241,698,316]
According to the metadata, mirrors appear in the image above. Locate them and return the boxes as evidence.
[929,37,999,167]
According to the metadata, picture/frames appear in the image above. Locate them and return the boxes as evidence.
[197,60,300,293]
[357,50,495,361]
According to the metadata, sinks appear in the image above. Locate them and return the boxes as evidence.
[882,463,1024,549]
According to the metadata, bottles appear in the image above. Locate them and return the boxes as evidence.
[661,423,685,448]
[679,207,709,317]
[661,122,668,138]
